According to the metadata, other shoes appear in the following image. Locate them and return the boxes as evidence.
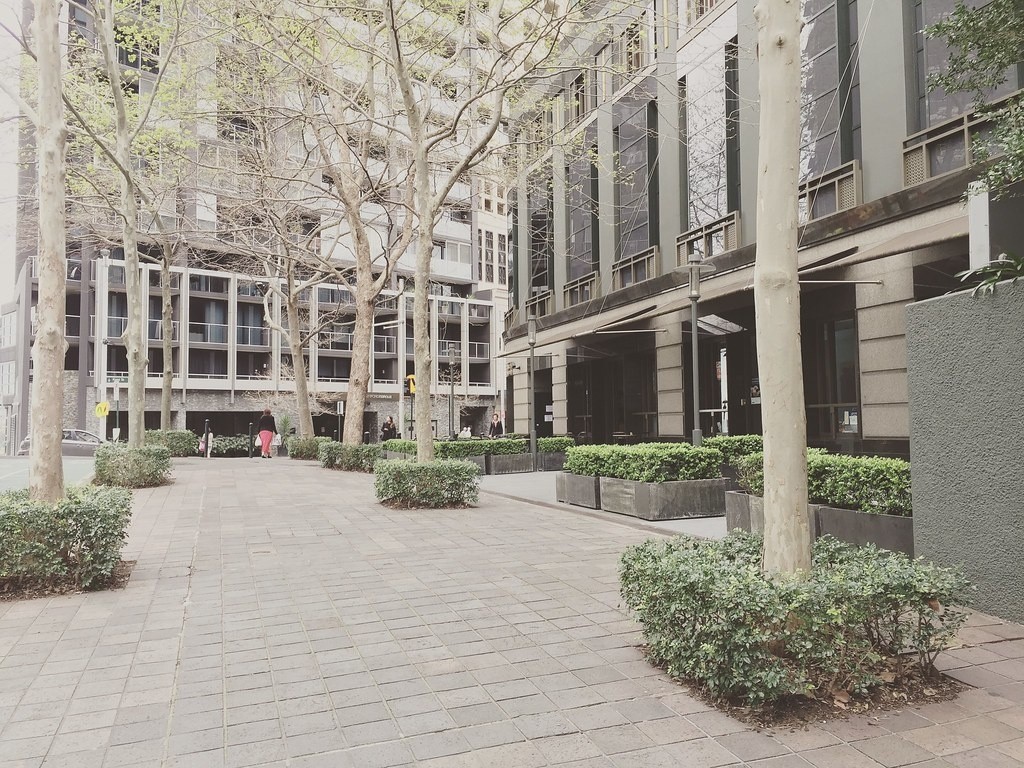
[268,455,272,458]
[262,455,266,458]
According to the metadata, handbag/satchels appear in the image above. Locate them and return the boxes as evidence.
[199,441,206,452]
[255,434,262,446]
[272,433,281,446]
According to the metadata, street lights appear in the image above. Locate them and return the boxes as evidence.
[526,315,537,473]
[673,254,716,445]
[447,342,456,442]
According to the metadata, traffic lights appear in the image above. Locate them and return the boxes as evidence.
[404,377,410,396]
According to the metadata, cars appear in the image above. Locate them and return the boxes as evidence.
[17,428,110,457]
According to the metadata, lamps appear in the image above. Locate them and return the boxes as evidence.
[507,362,520,370]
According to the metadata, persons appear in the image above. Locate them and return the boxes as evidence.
[258,409,278,458]
[382,416,396,441]
[460,425,472,437]
[202,427,213,458]
[488,414,503,438]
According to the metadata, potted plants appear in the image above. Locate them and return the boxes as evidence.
[276,414,290,456]
[378,432,914,561]
